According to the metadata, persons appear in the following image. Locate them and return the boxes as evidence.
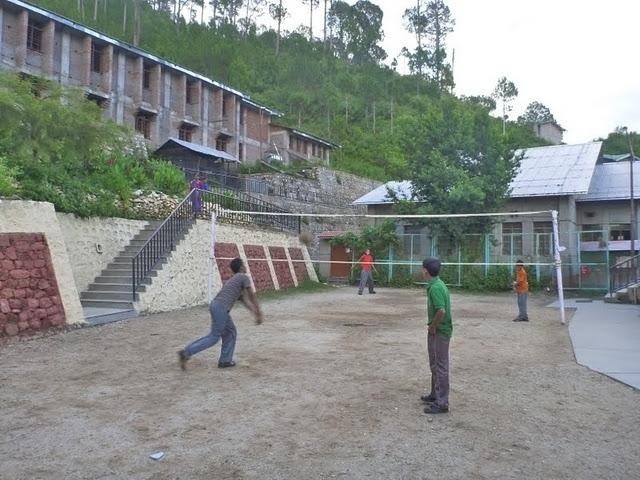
[510,259,529,322]
[189,172,205,216]
[175,257,263,369]
[419,256,455,414]
[348,248,380,295]
[198,176,209,190]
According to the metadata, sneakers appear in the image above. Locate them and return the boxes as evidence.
[423,404,448,414]
[218,361,235,367]
[369,291,375,294]
[421,395,436,402]
[177,350,189,370]
[513,317,528,321]
[359,291,362,295]
